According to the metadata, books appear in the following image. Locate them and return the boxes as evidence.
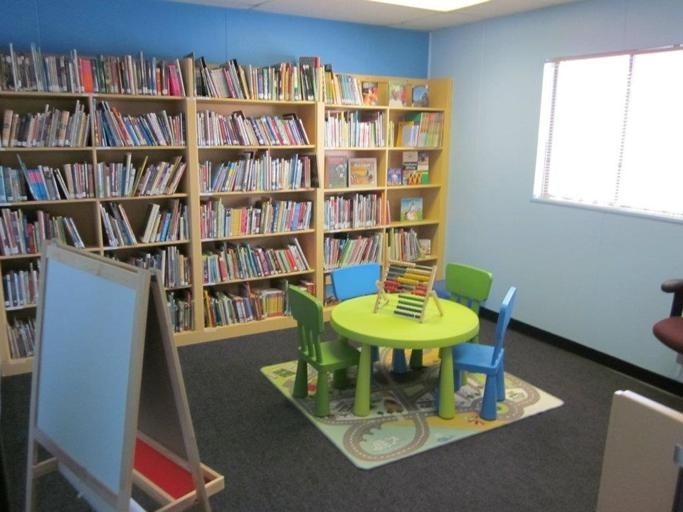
[320,64,442,307]
[1,152,189,256]
[1,42,187,148]
[197,148,313,238]
[202,235,317,326]
[3,258,42,359]
[188,52,320,146]
[105,245,193,334]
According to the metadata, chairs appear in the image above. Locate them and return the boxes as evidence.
[288,262,516,420]
[653,279,682,355]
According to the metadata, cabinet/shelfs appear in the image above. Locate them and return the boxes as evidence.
[0,52,453,378]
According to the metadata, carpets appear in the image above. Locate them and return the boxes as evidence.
[258,345,564,470]
